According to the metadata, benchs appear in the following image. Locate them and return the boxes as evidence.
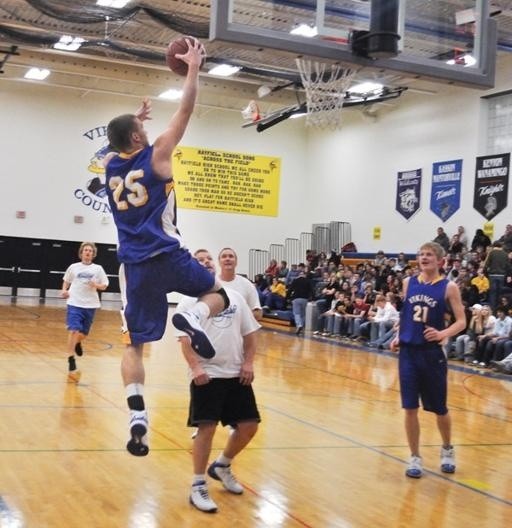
[253,256,512,373]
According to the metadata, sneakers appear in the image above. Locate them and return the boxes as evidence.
[439,444,457,473]
[449,351,512,375]
[73,342,83,356]
[171,309,217,361]
[187,480,219,513]
[294,325,364,342]
[404,454,424,479]
[68,356,77,371]
[206,461,245,495]
[125,410,151,458]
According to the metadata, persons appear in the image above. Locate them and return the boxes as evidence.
[169,248,261,512]
[59,242,110,370]
[219,248,262,319]
[390,242,467,478]
[101,38,231,457]
[435,224,512,378]
[253,245,411,354]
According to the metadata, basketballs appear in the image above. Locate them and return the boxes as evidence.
[166,36,205,76]
